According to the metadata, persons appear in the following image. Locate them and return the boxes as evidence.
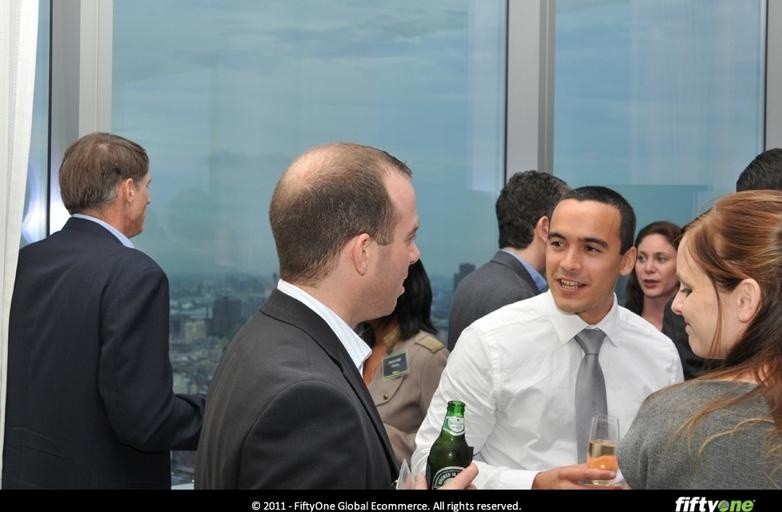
[613,190,781,491]
[624,220,722,383]
[1,130,209,490]
[355,259,450,471]
[447,171,569,359]
[405,184,683,491]
[193,139,484,490]
[730,146,781,191]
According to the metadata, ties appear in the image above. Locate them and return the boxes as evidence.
[576,329,610,465]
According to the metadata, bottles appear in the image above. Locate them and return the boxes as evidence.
[425,401,470,489]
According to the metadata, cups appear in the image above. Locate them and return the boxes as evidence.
[588,415,620,487]
